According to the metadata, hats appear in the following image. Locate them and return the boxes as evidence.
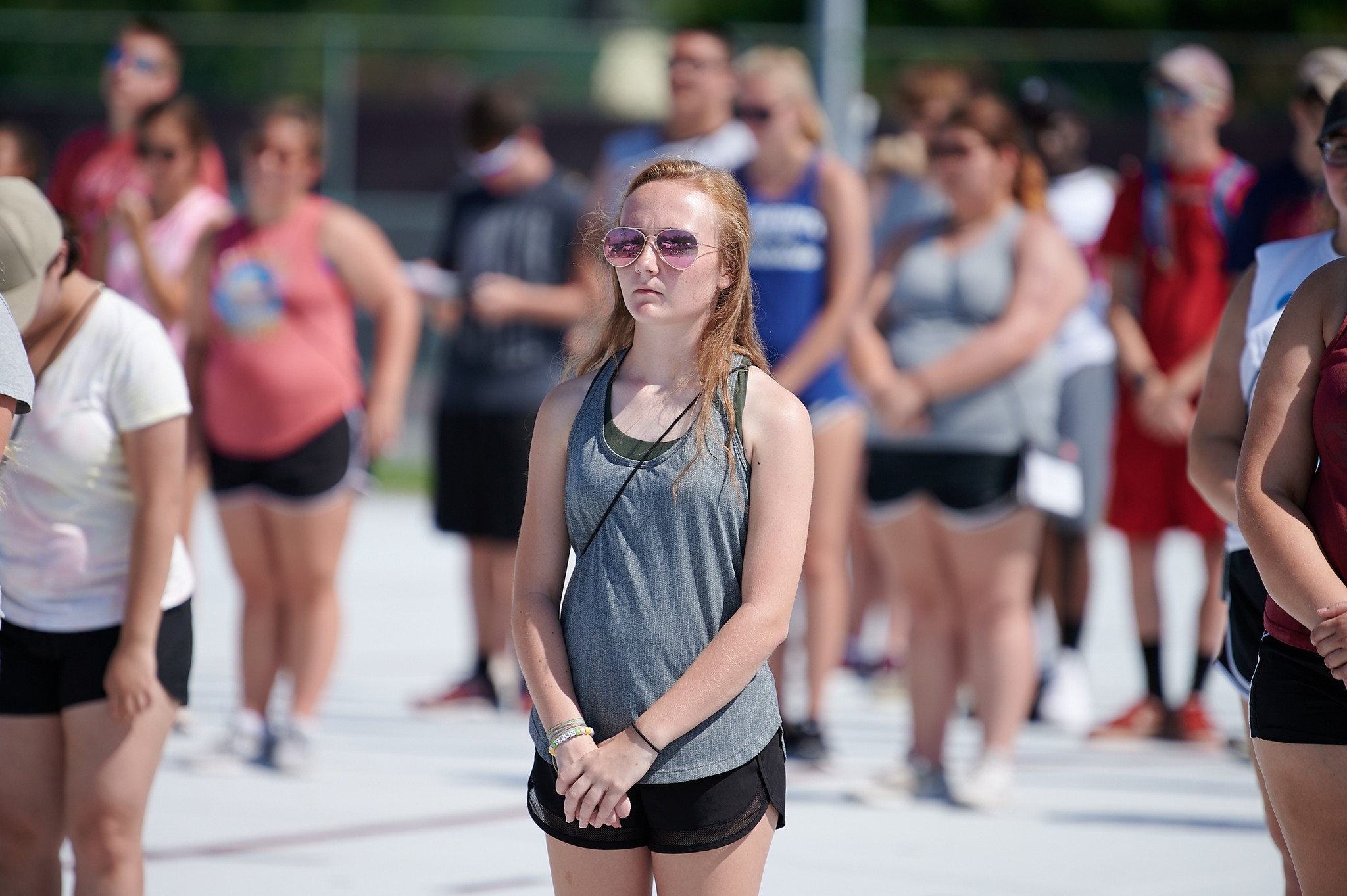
[0,176,64,333]
[1316,81,1347,145]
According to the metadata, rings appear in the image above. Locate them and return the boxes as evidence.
[592,807,598,813]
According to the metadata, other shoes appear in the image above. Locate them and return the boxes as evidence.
[413,672,503,710]
[269,730,320,782]
[1085,696,1218,742]
[182,728,262,782]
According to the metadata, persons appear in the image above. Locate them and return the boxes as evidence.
[0,174,196,895]
[0,24,759,769]
[509,160,815,896]
[726,39,1346,895]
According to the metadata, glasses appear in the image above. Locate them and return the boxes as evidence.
[1315,141,1347,167]
[601,227,718,271]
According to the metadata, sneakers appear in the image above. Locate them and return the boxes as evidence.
[788,728,1020,811]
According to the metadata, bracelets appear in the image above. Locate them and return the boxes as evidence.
[546,717,595,773]
[631,721,662,755]
